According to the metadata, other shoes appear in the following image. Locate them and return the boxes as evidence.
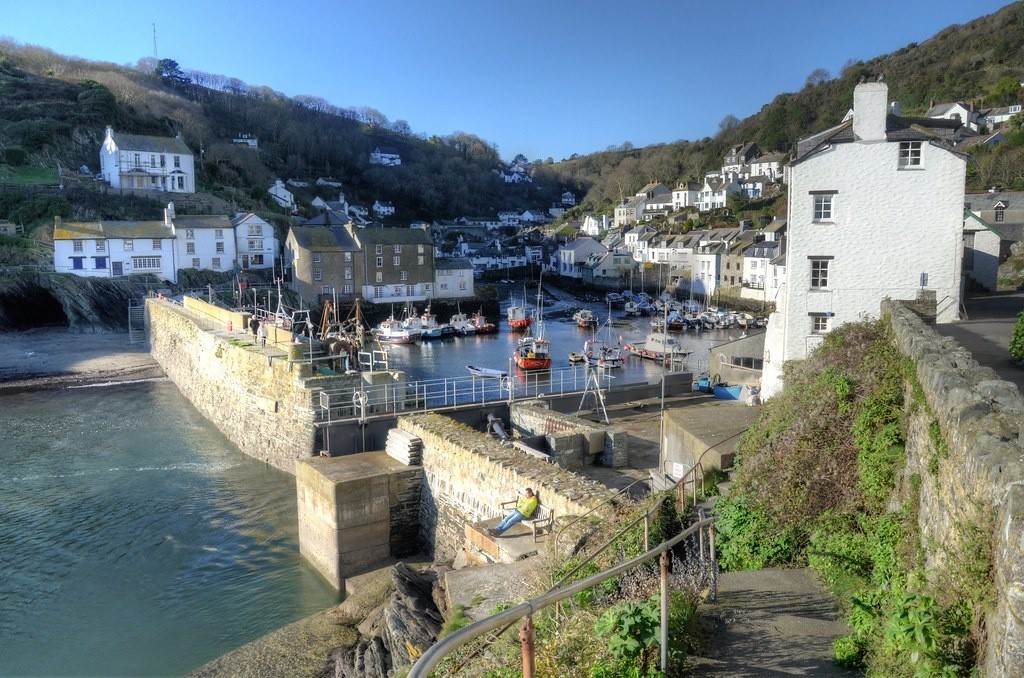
[487,528,499,538]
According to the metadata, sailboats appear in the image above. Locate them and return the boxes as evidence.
[606,258,769,329]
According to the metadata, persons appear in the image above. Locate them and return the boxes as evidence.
[257,322,269,345]
[487,488,537,537]
[250,315,260,343]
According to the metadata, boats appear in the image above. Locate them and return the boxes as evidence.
[568,352,583,362]
[575,305,599,326]
[582,301,628,368]
[507,283,535,330]
[465,365,507,378]
[370,297,495,344]
[624,328,694,362]
[514,272,553,369]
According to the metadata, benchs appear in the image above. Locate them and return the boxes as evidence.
[500,490,554,544]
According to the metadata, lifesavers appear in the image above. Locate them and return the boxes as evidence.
[333,340,351,356]
[322,337,338,352]
